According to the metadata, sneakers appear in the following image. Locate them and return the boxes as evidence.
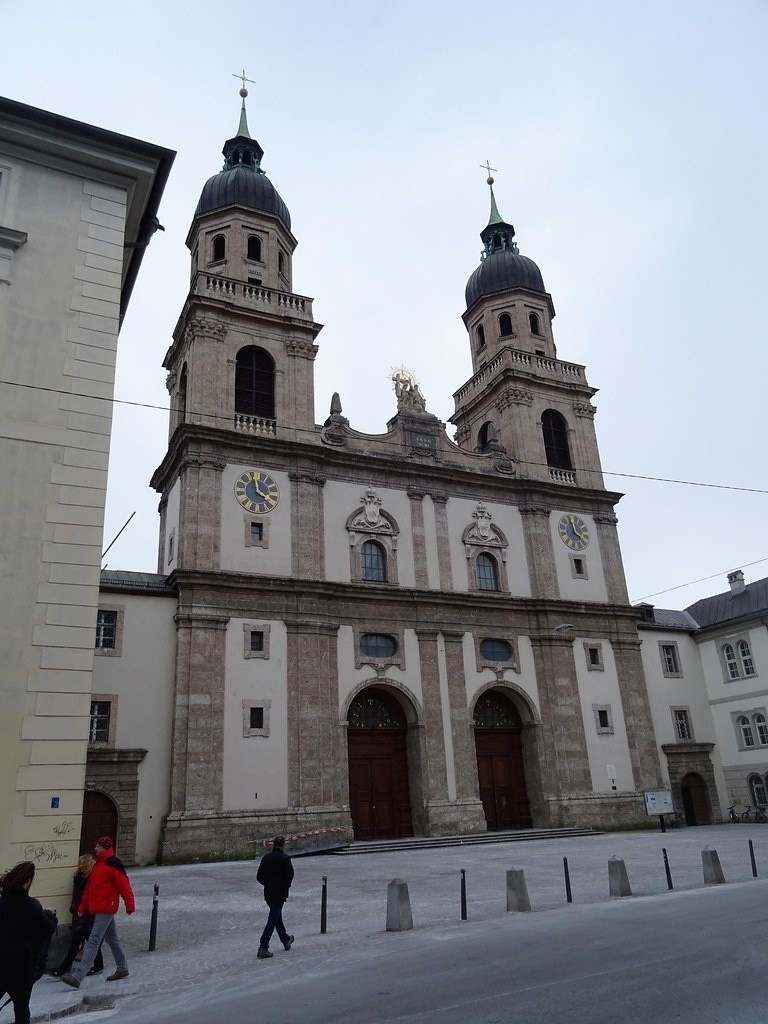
[107,970,130,981]
[283,935,294,951]
[257,947,274,959]
[61,974,80,989]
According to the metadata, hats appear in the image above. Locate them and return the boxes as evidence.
[96,836,113,850]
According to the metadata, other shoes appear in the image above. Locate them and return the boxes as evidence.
[47,968,71,977]
[86,967,103,976]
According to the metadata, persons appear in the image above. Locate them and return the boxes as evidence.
[0,861,56,1023]
[393,381,427,412]
[60,835,135,989]
[46,852,104,977]
[257,835,296,958]
